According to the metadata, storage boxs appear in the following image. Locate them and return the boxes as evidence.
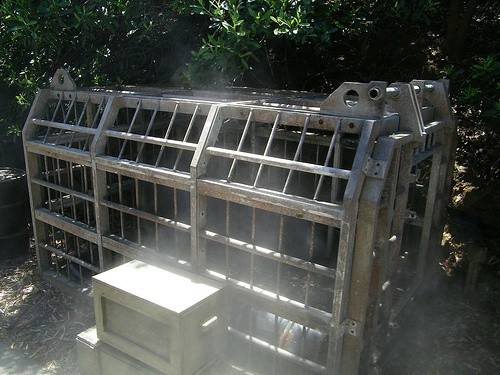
[75,323,102,375]
[93,259,229,374]
[100,344,165,375]
